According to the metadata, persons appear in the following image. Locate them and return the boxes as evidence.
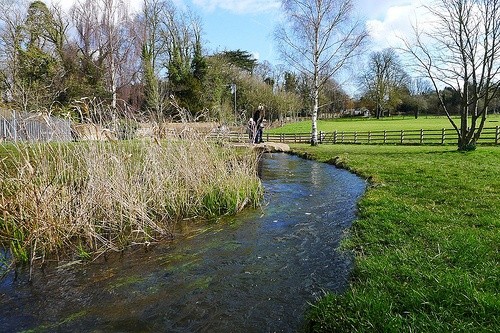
[254,104,266,144]
[248,117,258,143]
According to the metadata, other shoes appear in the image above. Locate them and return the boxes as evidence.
[255,140,264,144]
